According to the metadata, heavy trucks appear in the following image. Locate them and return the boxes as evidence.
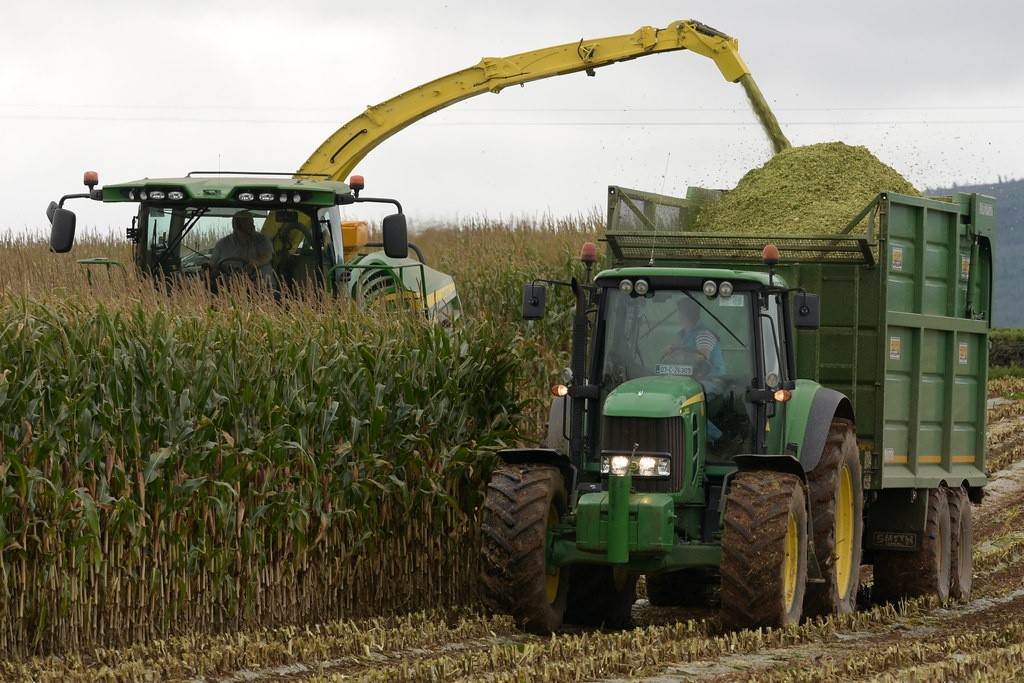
[477,184,999,634]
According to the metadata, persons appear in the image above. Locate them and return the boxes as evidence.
[662,297,738,465]
[210,211,281,307]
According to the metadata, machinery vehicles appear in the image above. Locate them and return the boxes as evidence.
[45,18,795,345]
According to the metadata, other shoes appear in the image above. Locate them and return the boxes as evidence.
[712,433,738,463]
